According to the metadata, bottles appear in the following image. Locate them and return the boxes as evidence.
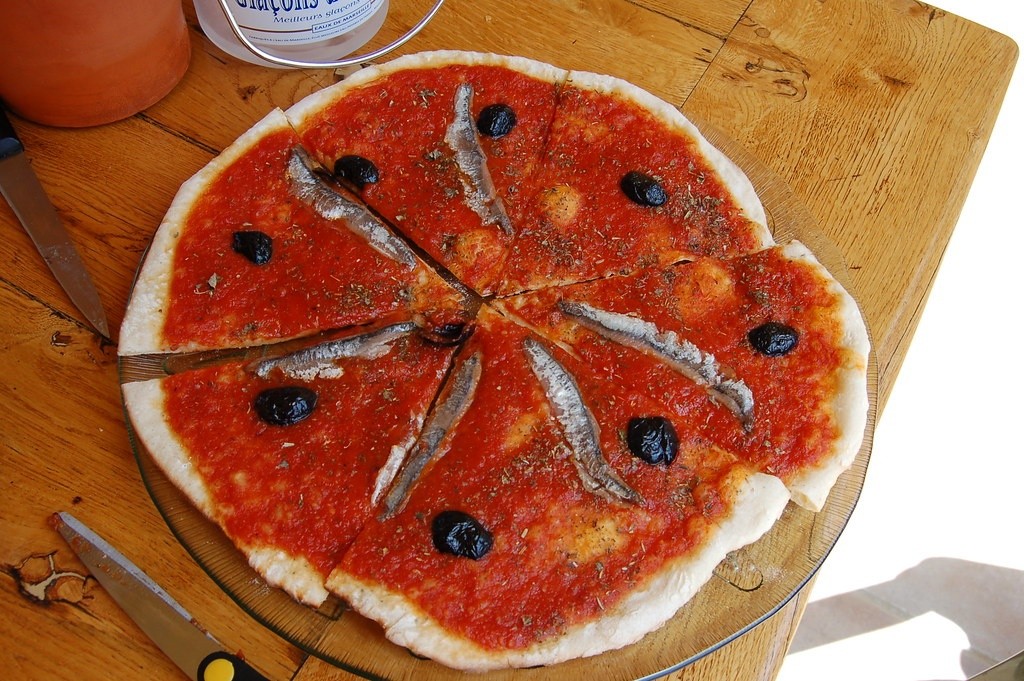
[0,0,191,127]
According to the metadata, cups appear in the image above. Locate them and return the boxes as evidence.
[193,0,389,68]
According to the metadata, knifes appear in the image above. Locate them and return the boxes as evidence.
[53,511,271,681]
[0,106,109,339]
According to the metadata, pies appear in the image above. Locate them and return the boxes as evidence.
[118,49,871,673]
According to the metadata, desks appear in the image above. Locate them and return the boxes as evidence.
[0,0,1018,681]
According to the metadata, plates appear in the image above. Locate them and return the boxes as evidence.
[124,107,877,681]
